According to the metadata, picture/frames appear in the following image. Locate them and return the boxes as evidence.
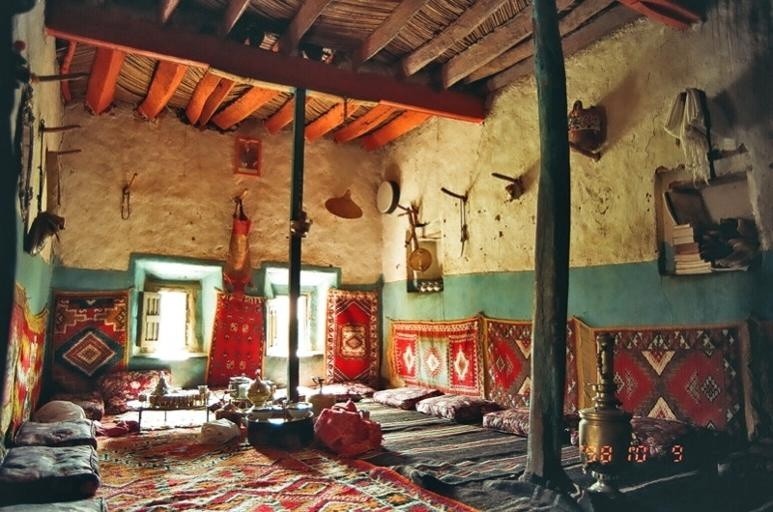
[234,136,262,177]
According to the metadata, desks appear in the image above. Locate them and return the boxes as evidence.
[127,388,317,447]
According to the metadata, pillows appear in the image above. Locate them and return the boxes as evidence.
[0,367,172,512]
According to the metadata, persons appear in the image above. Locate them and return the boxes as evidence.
[240,142,258,169]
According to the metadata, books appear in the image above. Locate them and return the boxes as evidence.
[663,191,752,275]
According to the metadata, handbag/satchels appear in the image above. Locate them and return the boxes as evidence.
[27,212,65,256]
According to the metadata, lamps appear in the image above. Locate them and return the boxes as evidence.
[322,188,363,221]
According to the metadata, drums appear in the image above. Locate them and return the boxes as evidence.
[376,182,399,214]
